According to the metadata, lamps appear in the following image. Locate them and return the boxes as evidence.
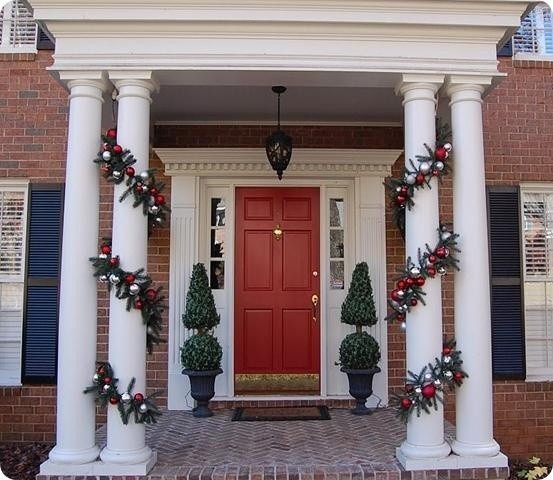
[263,85,295,181]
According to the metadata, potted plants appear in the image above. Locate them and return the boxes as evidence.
[178,259,383,417]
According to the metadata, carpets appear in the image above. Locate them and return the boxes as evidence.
[232,407,331,422]
